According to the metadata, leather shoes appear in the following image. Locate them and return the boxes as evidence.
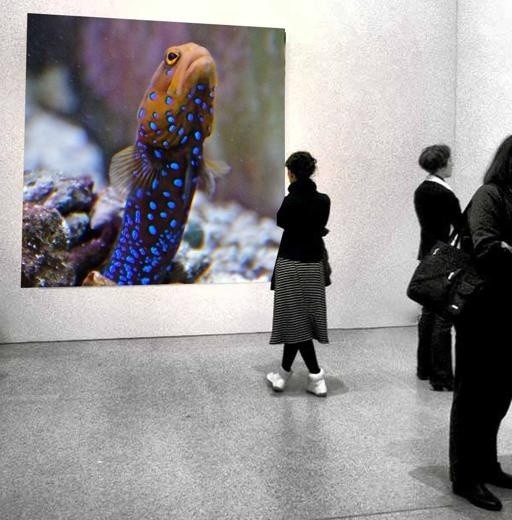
[453,482,502,510]
[487,463,511,488]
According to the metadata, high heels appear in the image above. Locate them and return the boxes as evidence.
[431,376,454,391]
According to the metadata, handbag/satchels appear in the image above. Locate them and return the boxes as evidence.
[407,240,484,320]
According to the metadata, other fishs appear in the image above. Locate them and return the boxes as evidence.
[101,42,233,285]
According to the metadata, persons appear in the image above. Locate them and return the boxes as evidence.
[410,143,463,394]
[447,135,511,512]
[261,151,333,401]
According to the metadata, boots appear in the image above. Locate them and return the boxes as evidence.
[307,368,327,397]
[266,366,292,391]
